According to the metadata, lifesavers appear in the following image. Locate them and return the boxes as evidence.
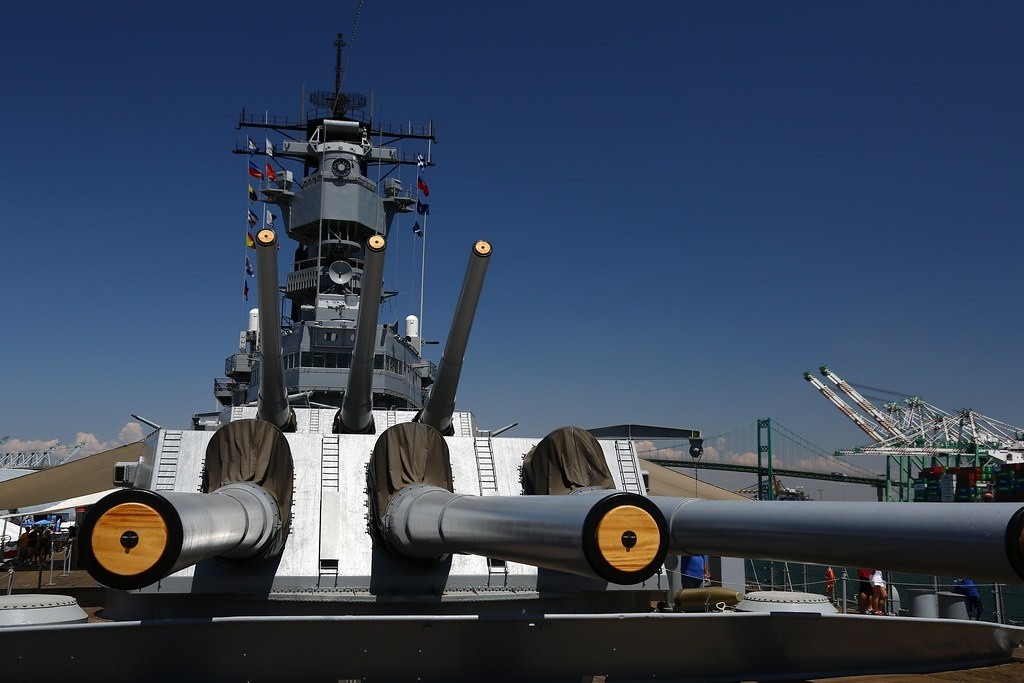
[824,568,836,594]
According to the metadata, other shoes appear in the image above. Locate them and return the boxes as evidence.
[855,604,887,616]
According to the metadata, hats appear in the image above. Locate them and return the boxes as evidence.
[34,528,40,530]
[67,526,76,529]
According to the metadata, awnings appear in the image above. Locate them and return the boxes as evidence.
[0,487,130,519]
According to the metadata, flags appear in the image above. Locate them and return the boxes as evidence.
[244,135,280,301]
[412,152,430,237]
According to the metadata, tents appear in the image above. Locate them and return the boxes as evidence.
[20,520,55,525]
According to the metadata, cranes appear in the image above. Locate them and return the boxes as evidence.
[803,365,1023,505]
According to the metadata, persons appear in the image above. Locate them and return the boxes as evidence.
[858,569,887,614]
[17,525,51,566]
[66,526,78,570]
[955,578,983,620]
[680,553,709,589]
